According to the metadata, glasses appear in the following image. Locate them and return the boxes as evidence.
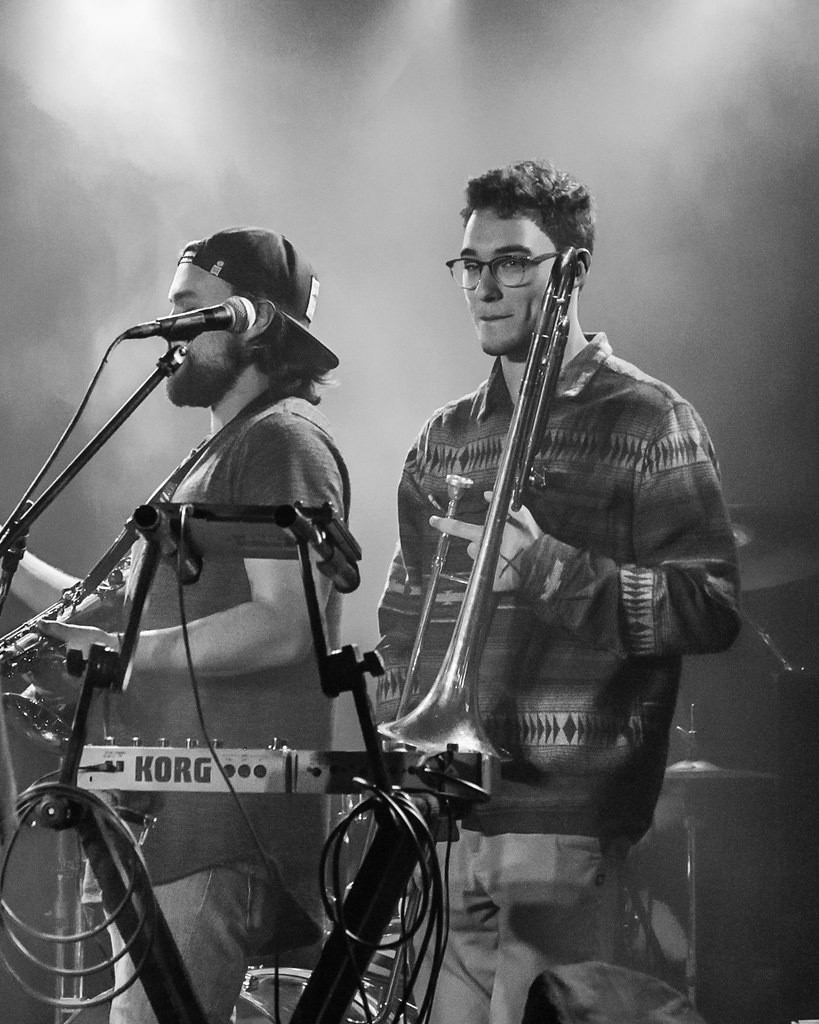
[445,251,561,290]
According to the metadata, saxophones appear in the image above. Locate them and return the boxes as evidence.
[0,495,165,756]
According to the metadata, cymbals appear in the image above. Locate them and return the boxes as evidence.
[725,501,819,595]
[663,758,781,784]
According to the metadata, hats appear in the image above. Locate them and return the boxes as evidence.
[177,226,339,376]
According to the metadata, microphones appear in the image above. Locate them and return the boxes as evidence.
[122,296,255,339]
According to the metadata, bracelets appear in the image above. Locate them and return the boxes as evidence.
[115,630,122,653]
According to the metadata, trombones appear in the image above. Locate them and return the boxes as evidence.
[336,242,582,1022]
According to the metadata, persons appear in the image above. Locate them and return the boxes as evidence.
[373,156,745,1024]
[0,227,350,1023]
